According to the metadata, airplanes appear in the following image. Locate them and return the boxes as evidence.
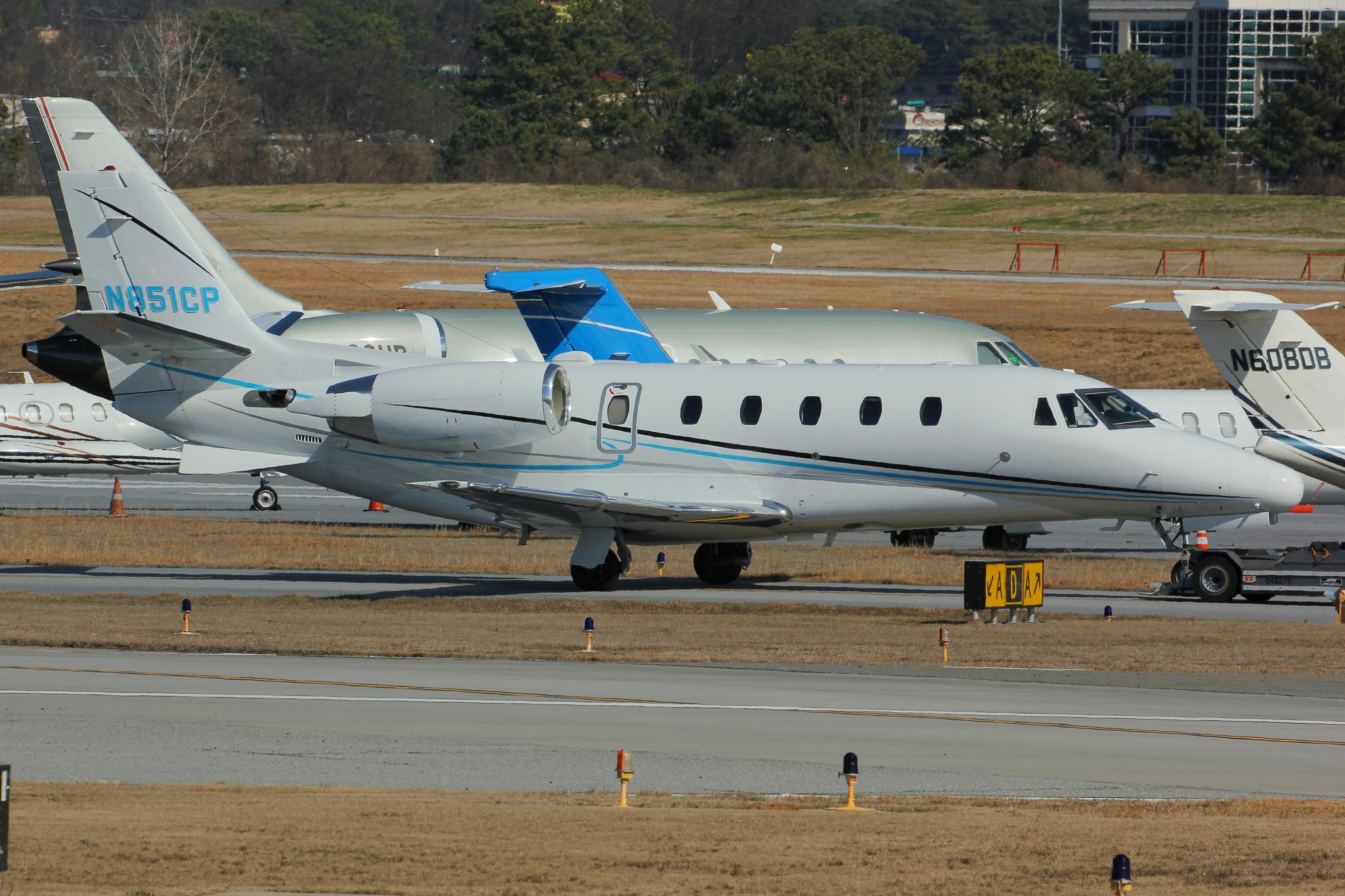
[19,96,1345,590]
